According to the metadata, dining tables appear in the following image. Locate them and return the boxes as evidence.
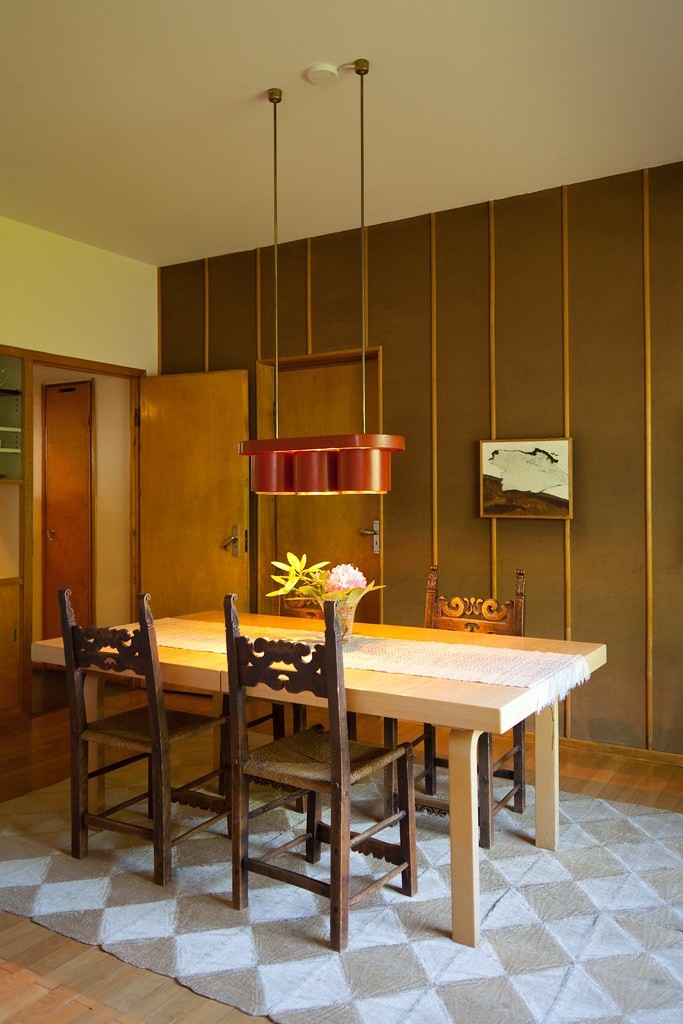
[383,565,527,849]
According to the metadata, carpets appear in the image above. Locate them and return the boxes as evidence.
[0,721,683,1024]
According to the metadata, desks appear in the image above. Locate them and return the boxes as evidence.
[32,610,607,947]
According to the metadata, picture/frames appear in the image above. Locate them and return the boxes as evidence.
[480,436,573,519]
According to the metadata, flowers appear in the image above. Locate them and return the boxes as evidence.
[266,552,386,635]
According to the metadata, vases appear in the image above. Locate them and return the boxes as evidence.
[318,597,359,649]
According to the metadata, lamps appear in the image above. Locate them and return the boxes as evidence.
[237,56,405,495]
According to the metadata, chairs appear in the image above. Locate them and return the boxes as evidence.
[59,587,233,886]
[230,586,385,812]
[223,592,418,952]
